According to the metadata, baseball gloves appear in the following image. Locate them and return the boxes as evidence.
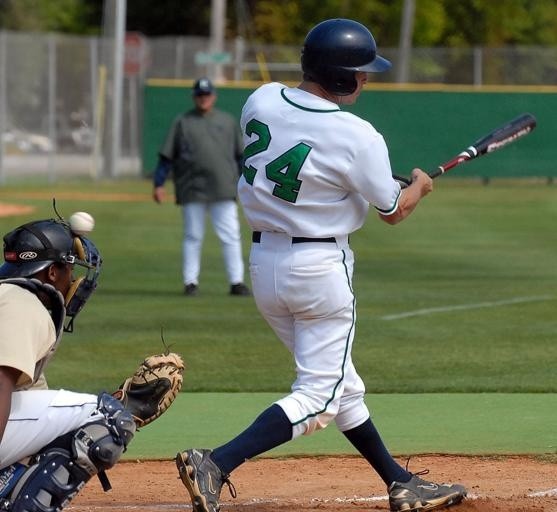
[111,352,185,429]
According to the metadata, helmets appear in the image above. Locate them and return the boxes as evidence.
[1,217,73,279]
[301,17,392,97]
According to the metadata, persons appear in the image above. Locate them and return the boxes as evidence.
[0,216,184,512]
[175,20,468,511]
[151,78,251,297]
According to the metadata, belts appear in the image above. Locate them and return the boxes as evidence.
[252,230,337,244]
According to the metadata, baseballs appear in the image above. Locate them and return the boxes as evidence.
[68,212,95,235]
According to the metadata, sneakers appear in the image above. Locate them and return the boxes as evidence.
[387,473,468,512]
[175,446,227,512]
[185,284,198,295]
[230,284,252,296]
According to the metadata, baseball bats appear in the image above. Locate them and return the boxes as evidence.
[410,113,538,185]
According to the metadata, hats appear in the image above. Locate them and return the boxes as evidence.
[193,78,215,95]
